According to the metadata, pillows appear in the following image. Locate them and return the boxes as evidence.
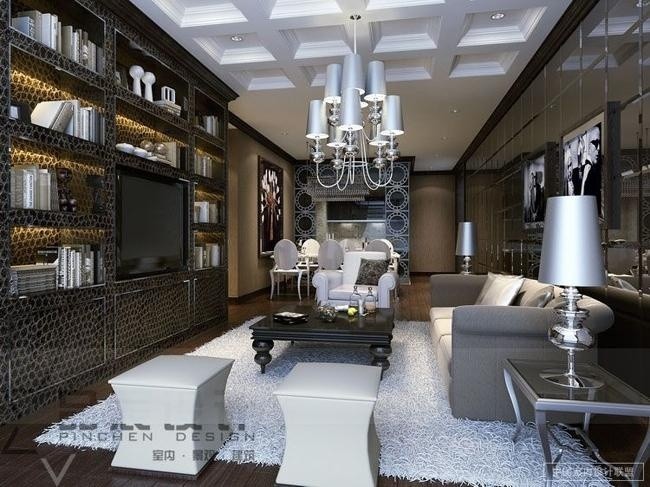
[524,285,554,307]
[479,275,525,306]
[355,258,392,285]
[475,271,523,306]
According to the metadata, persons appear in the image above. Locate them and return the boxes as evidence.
[529,169,542,219]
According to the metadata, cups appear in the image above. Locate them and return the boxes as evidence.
[311,301,321,318]
[317,300,331,318]
[323,303,336,322]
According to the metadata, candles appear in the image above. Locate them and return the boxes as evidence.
[325,233,328,239]
[332,233,334,240]
[328,234,330,238]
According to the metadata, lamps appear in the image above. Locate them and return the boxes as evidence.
[455,222,478,275]
[306,13,405,190]
[539,196,606,388]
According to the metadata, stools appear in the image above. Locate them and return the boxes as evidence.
[107,355,234,477]
[272,363,381,486]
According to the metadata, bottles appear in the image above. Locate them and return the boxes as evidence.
[350,286,359,311]
[364,287,377,315]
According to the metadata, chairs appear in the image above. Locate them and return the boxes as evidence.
[319,240,345,270]
[302,239,320,257]
[365,240,390,252]
[270,240,304,301]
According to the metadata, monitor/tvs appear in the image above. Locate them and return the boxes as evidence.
[117,164,189,281]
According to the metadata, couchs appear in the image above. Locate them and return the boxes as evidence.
[312,251,398,309]
[431,274,615,424]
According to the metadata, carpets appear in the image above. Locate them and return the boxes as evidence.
[36,319,615,487]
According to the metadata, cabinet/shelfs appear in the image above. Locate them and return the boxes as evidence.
[0,0,238,421]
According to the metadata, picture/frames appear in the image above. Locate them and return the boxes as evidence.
[560,102,622,229]
[257,155,284,255]
[521,143,558,229]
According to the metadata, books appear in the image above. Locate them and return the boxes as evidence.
[12,164,59,212]
[197,112,223,138]
[195,152,225,182]
[194,200,224,223]
[12,244,105,295]
[195,243,225,267]
[13,9,103,72]
[31,97,104,144]
[165,141,187,170]
